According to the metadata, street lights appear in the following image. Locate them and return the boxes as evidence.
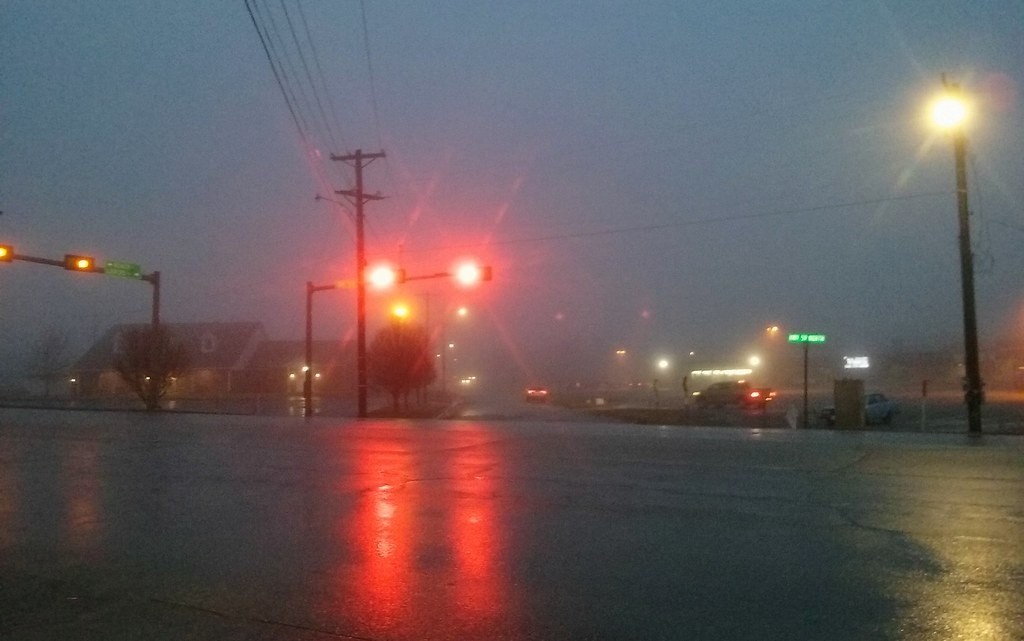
[926,72,987,439]
[658,360,668,420]
[392,303,409,416]
[788,333,825,428]
[422,290,467,404]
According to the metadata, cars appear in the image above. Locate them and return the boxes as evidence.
[945,320,1024,389]
[525,385,548,403]
[821,392,892,429]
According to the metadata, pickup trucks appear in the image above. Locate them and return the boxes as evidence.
[693,380,773,408]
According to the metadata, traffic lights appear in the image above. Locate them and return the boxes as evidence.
[65,255,97,272]
[366,269,405,290]
[0,244,15,262]
[456,266,493,285]
